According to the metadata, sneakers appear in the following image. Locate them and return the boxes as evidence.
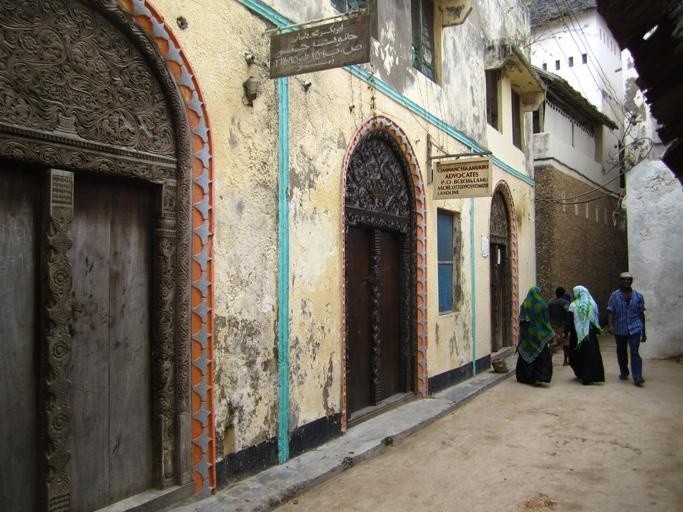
[619,375,645,386]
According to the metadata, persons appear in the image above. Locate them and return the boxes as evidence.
[562,291,571,365]
[514,285,556,386]
[606,271,647,387]
[562,285,604,384]
[546,286,571,366]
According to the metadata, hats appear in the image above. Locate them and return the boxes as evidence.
[618,271,633,279]
